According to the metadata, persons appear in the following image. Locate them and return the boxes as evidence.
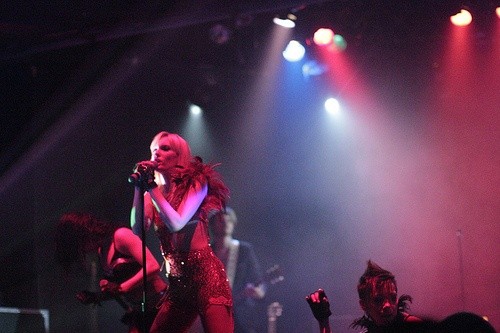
[131,131,234,333]
[209,207,265,333]
[55,212,169,333]
[305,260,497,333]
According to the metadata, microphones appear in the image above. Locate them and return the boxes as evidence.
[127,160,158,183]
[99,279,135,311]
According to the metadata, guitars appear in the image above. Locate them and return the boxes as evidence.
[234,264,284,302]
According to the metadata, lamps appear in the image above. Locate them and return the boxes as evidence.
[273,9,299,29]
